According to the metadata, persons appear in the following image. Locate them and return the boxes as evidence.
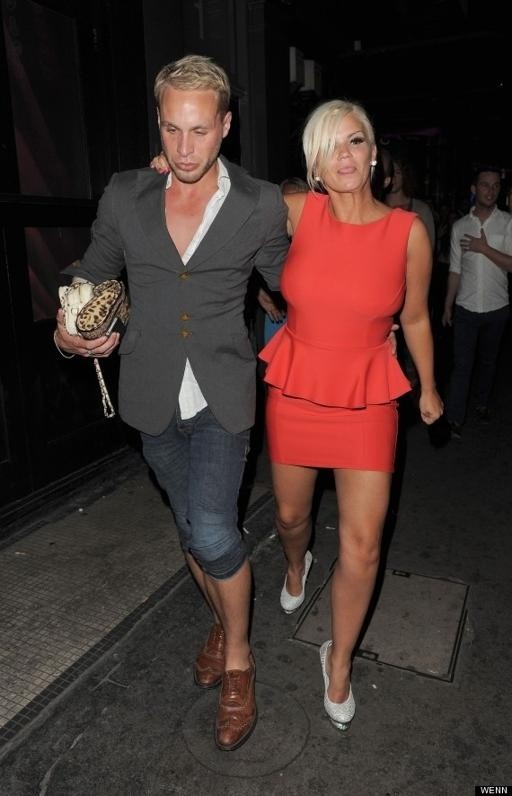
[144,96,445,735]
[44,51,404,757]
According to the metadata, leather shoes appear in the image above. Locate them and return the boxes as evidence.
[192,621,228,689]
[213,646,259,753]
[318,639,357,731]
[278,548,314,616]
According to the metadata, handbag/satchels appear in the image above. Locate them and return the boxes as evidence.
[57,277,130,358]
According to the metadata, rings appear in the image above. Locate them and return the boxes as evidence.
[86,347,96,355]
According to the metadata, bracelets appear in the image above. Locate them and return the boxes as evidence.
[51,329,78,362]
